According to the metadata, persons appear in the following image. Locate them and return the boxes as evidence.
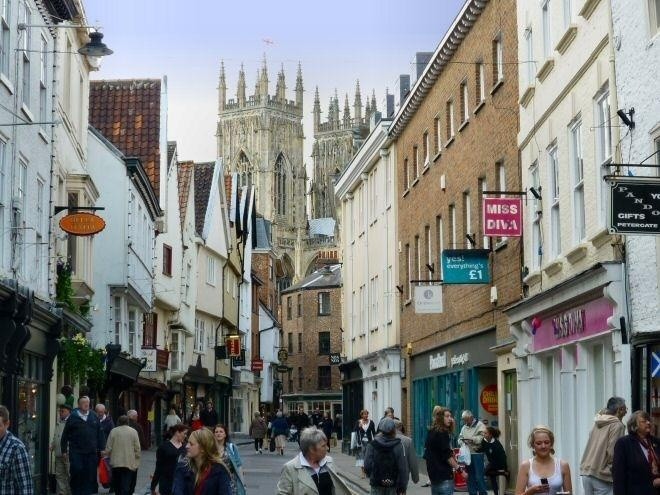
[0,404,34,494]
[470,426,510,495]
[355,407,419,495]
[16,404,36,455]
[421,405,443,489]
[580,396,626,495]
[54,393,247,495]
[275,424,353,495]
[249,408,342,456]
[514,425,572,495]
[611,409,660,495]
[422,405,469,495]
[457,410,492,495]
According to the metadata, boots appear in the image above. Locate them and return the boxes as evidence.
[360,467,366,479]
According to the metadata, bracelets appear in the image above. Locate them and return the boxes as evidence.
[456,466,464,474]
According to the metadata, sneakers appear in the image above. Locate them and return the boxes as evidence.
[259,449,262,454]
[281,449,283,456]
[256,451,259,454]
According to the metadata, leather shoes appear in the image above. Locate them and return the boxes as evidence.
[420,483,431,487]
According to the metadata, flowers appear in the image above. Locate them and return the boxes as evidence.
[58,332,107,386]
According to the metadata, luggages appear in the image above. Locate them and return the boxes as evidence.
[49,449,56,493]
[290,425,297,442]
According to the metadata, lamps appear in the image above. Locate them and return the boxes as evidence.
[19,22,111,58]
[319,262,343,281]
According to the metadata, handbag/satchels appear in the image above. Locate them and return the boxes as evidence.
[328,438,334,448]
[351,432,357,450]
[270,438,275,452]
[102,458,112,488]
[332,437,337,447]
[99,456,109,485]
[264,432,268,450]
